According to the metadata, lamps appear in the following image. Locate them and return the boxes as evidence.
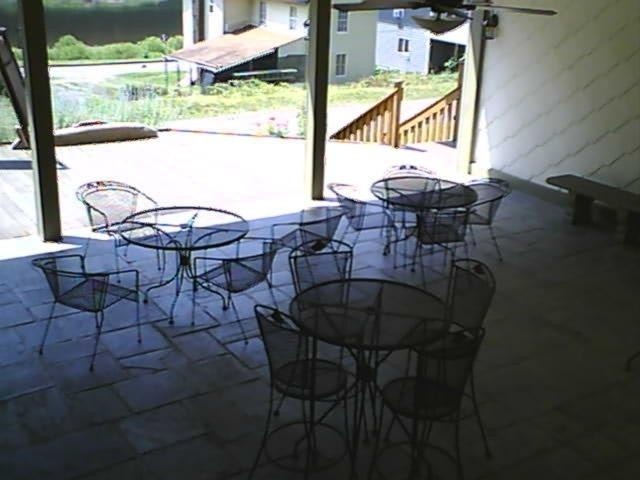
[480,9,499,40]
[408,6,468,36]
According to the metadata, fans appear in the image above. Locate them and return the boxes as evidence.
[332,0,557,36]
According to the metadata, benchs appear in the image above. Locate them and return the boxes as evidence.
[546,172,640,242]
